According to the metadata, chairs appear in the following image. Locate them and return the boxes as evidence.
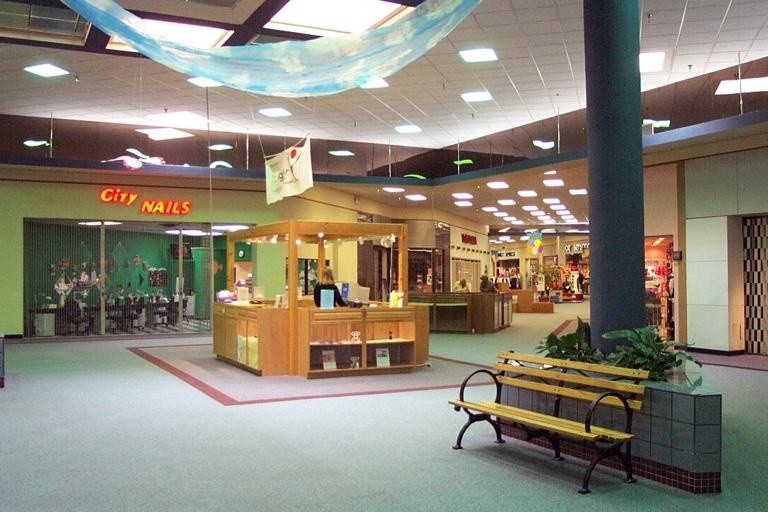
[62,297,189,335]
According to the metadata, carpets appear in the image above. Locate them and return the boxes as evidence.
[124,343,524,405]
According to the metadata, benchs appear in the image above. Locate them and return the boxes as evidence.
[447,350,651,496]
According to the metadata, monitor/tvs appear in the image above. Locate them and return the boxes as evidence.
[340,281,369,302]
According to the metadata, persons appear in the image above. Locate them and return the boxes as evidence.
[458,279,470,292]
[314,268,347,308]
[480,275,497,291]
